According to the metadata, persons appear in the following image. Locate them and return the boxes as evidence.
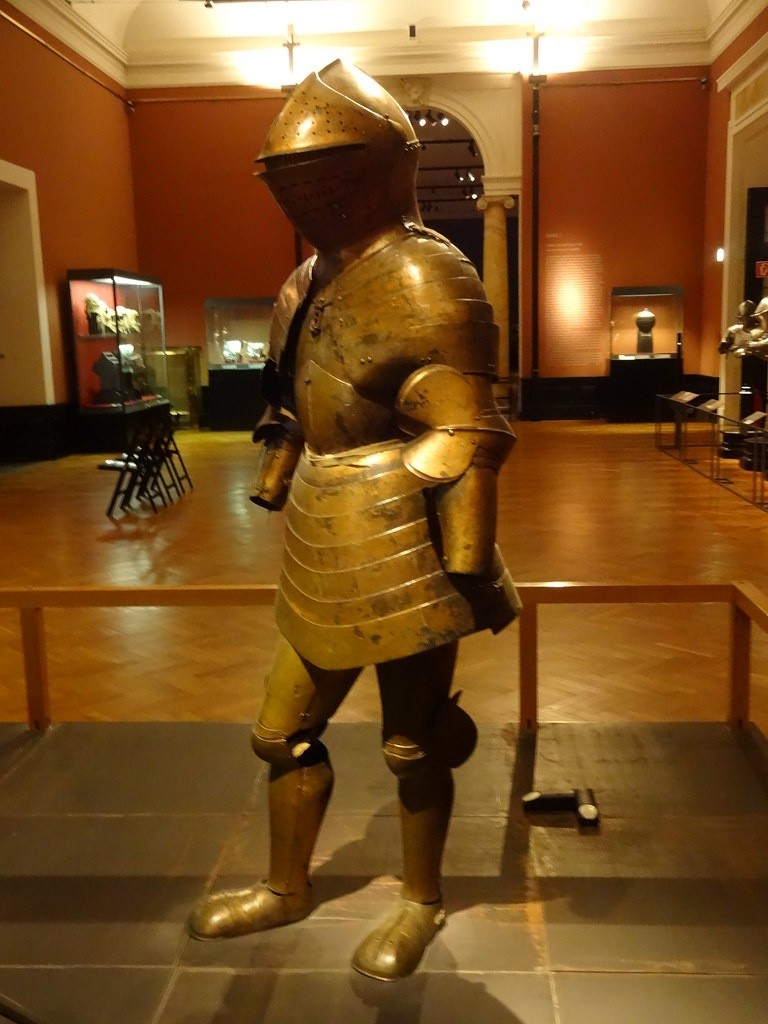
[185,60,524,982]
[718,296,768,358]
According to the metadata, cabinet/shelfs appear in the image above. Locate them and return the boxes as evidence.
[62,268,172,453]
